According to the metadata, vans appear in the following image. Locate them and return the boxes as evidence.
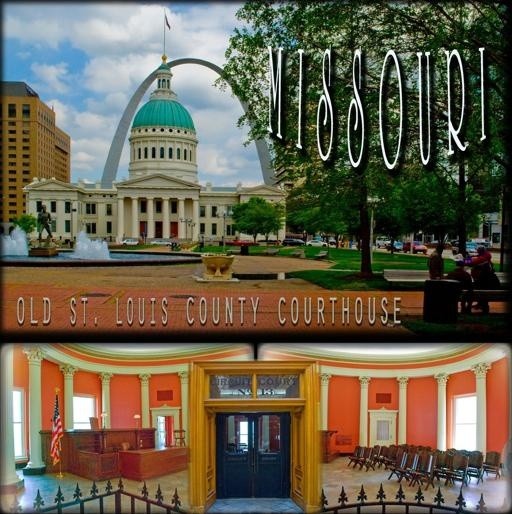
[283,239,305,246]
[376,236,391,248]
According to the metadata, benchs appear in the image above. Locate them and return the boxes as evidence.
[459,282,505,312]
[289,249,304,256]
[263,249,279,255]
[383,269,508,283]
[315,250,328,258]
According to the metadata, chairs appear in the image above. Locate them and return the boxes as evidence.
[347,444,501,491]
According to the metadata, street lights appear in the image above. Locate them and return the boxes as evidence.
[179,216,193,241]
[188,221,195,239]
[367,195,386,261]
[303,230,307,245]
[216,210,234,252]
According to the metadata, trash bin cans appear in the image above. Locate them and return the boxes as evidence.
[240,245,249,255]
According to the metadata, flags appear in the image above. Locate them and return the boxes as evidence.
[163,8,172,31]
[50,386,66,467]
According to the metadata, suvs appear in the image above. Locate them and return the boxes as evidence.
[451,241,478,257]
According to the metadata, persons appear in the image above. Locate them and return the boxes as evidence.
[37,204,54,243]
[426,242,501,315]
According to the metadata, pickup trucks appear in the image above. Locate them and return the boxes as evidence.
[322,237,336,245]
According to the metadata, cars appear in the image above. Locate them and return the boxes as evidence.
[151,239,172,246]
[121,239,139,245]
[403,240,428,254]
[306,240,327,247]
[386,241,403,251]
